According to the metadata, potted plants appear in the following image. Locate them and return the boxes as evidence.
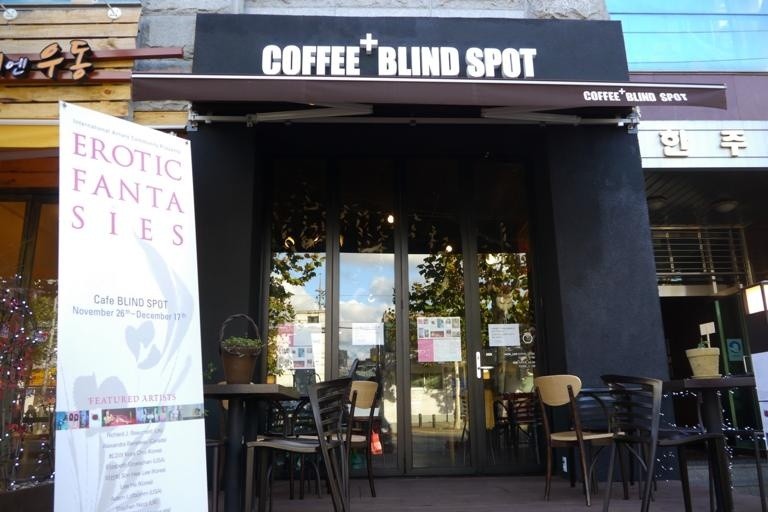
[686,334,723,379]
[218,313,264,384]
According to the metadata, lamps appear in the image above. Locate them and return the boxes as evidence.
[740,280,768,318]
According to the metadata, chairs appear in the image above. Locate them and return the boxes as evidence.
[484,369,768,512]
[204,357,384,512]
